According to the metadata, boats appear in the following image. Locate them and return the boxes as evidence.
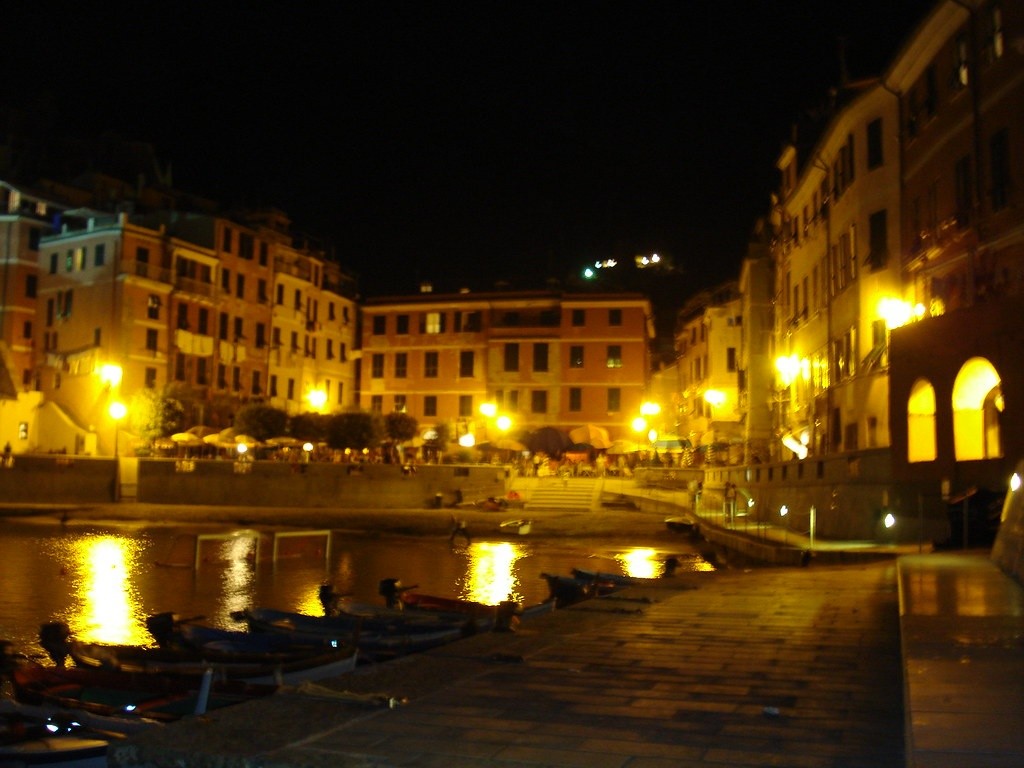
[519,596,560,618]
[663,512,694,532]
[0,637,223,744]
[321,583,470,635]
[39,617,284,707]
[146,610,361,679]
[570,563,639,585]
[226,606,464,664]
[539,572,620,605]
[498,517,532,536]
[0,680,127,765]
[378,574,522,631]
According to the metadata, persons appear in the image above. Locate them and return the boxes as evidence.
[490,448,674,477]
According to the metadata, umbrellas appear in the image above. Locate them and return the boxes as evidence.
[156,426,327,459]
[472,424,638,454]
